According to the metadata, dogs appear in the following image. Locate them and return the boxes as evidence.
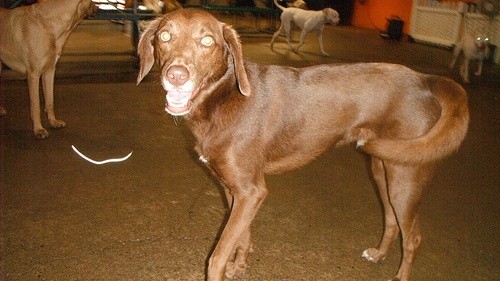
[136,7,469,281]
[449,31,489,84]
[269,0,340,57]
[0,0,99,140]
